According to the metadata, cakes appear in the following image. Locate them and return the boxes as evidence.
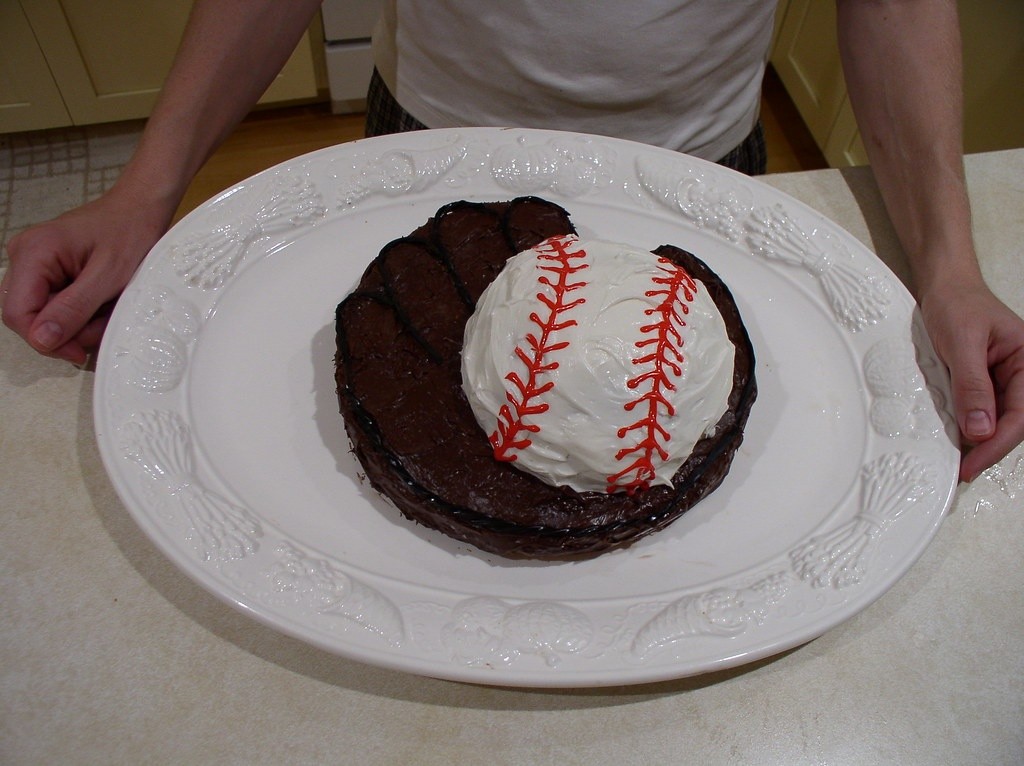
[337,195,757,561]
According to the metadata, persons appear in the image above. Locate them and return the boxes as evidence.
[0,0,1024,482]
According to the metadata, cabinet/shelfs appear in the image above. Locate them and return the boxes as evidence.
[774,0,1024,171]
[0,0,326,150]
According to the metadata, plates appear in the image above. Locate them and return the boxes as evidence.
[91,126,960,689]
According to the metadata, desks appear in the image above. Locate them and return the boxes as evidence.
[0,151,1024,766]
